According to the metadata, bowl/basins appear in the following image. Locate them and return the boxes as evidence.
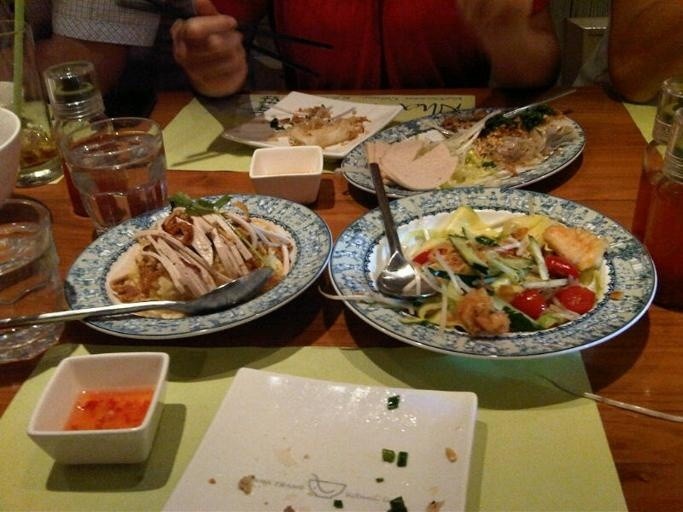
[26,349,172,467]
[248,145,326,204]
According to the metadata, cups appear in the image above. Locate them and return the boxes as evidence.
[0,194,66,364]
[0,18,66,190]
[60,115,169,238]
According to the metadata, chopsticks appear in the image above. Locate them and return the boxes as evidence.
[114,1,335,78]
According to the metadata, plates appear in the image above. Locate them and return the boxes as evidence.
[327,185,660,362]
[155,365,479,511]
[219,90,404,160]
[340,104,587,199]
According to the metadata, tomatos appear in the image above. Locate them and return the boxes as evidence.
[555,286,595,314]
[545,255,579,280]
[509,290,544,320]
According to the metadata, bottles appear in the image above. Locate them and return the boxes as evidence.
[629,74,683,311]
[42,59,129,219]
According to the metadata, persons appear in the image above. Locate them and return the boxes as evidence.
[169,0,566,103]
[606,1,683,105]
[0,1,166,101]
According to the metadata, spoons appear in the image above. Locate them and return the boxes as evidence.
[0,265,276,329]
[367,160,440,300]
